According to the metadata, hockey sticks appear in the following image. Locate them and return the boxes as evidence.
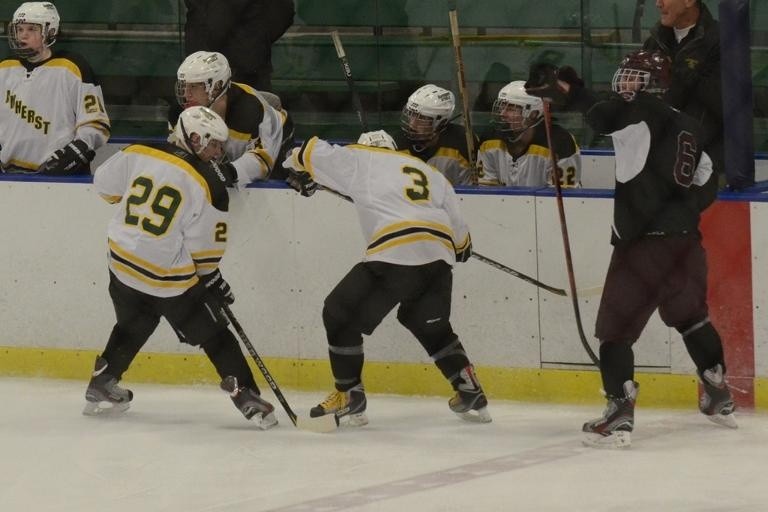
[539,93,603,368]
[314,180,605,300]
[222,302,340,430]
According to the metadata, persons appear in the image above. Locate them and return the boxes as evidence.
[524,46,740,437]
[389,83,474,185]
[183,0,298,94]
[640,1,726,173]
[470,74,580,191]
[84,104,280,418]
[1,1,112,175]
[167,50,294,188]
[281,128,491,417]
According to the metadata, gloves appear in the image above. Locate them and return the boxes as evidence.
[200,268,236,304]
[527,63,585,114]
[213,163,239,188]
[40,139,96,177]
[300,171,318,198]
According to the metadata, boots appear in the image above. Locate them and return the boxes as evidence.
[84,356,134,403]
[582,381,637,433]
[699,364,735,415]
[220,375,274,417]
[313,382,365,418]
[449,362,486,415]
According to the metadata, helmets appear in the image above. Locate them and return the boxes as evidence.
[7,2,59,63]
[169,106,229,158]
[491,81,546,142]
[401,83,456,141]
[356,129,399,151]
[174,51,232,109]
[609,48,675,101]
